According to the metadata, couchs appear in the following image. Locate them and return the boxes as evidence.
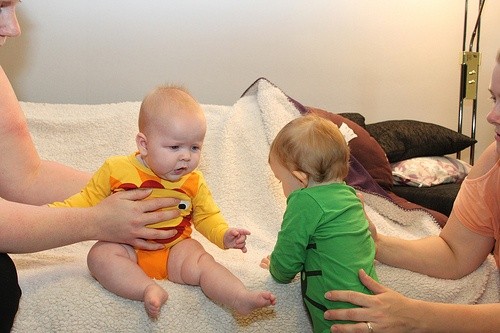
[6,105,449,333]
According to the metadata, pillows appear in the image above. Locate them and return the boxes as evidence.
[335,113,478,187]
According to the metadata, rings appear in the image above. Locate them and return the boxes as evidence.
[366,322,373,333]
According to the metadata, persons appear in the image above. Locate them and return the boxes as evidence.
[324,51,500,333]
[0,0,182,333]
[41,86,276,319]
[260,112,381,333]
